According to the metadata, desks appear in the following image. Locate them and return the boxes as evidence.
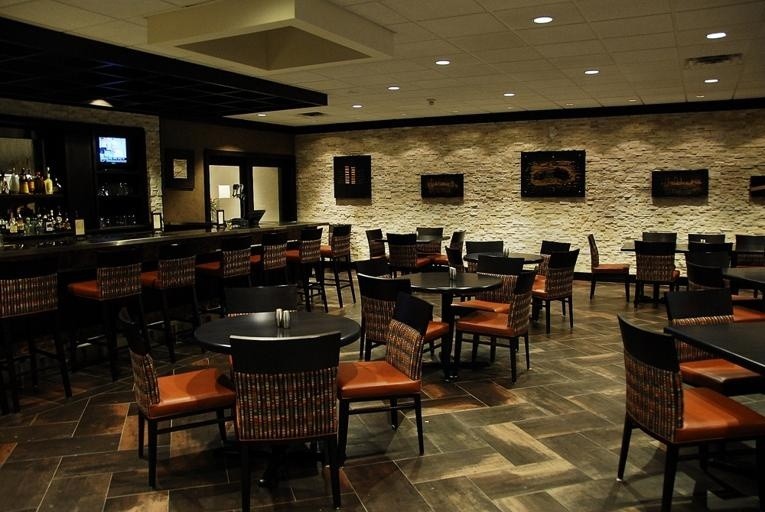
[621,241,765,254]
[3,221,329,279]
[194,311,361,353]
[463,252,545,264]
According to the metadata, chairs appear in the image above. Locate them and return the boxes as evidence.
[617,314,764,510]
[366,227,467,276]
[196,248,252,318]
[334,296,434,464]
[0,272,72,411]
[69,263,146,381]
[587,234,631,302]
[357,273,449,362]
[138,254,206,363]
[229,332,343,510]
[464,241,504,253]
[250,228,328,315]
[223,284,298,312]
[634,232,765,309]
[450,270,536,383]
[531,240,580,337]
[478,255,525,273]
[320,223,356,308]
[119,307,235,486]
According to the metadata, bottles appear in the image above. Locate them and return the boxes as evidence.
[0,167,54,195]
[275,308,282,327]
[282,310,290,329]
[0,208,71,237]
[448,266,457,281]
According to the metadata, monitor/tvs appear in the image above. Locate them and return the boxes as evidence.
[96,135,130,165]
